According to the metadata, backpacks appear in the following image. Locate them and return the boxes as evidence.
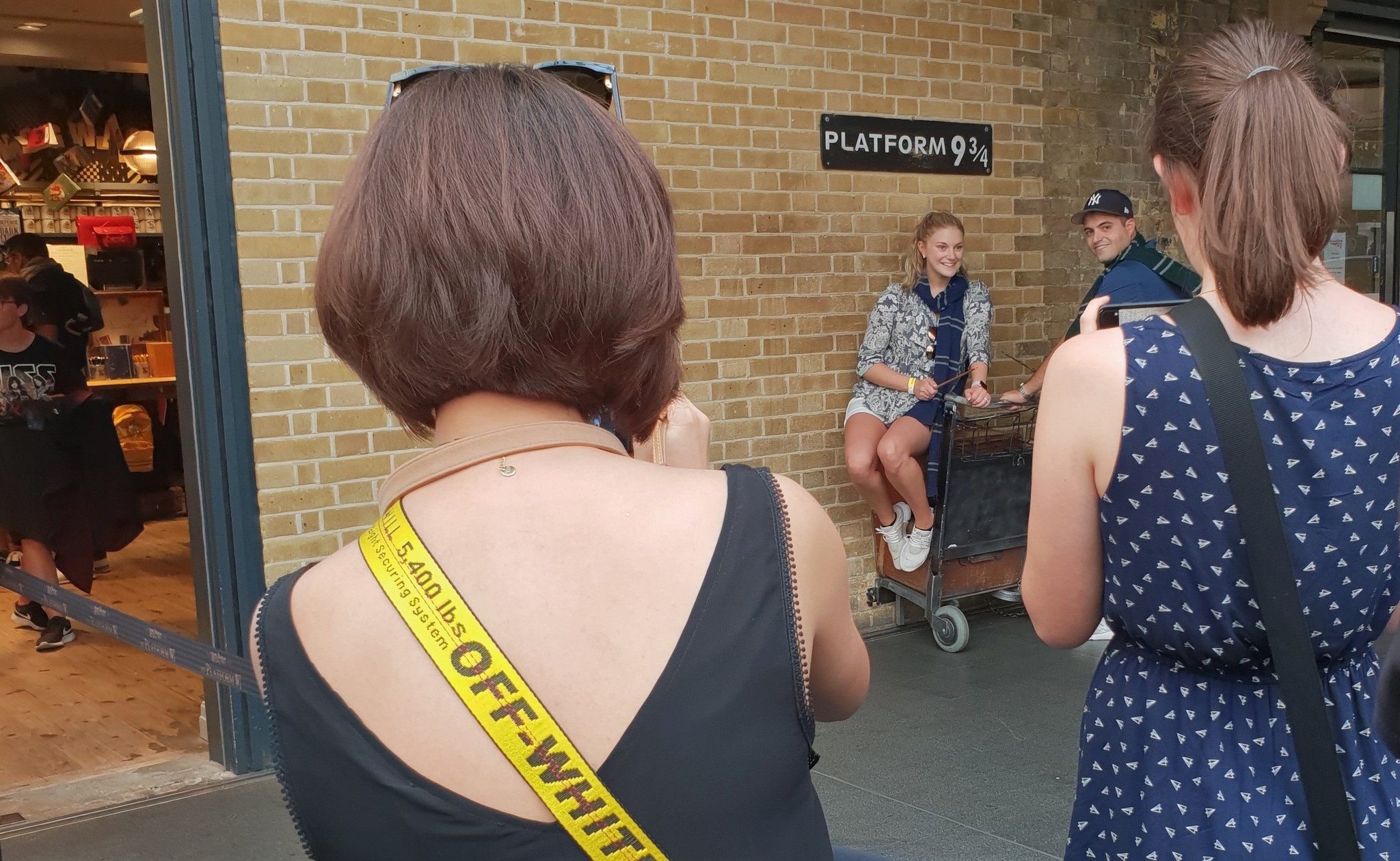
[112,404,154,471]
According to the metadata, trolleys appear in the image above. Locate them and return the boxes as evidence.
[864,401,1038,655]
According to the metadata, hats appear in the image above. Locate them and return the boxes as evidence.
[1070,189,1133,224]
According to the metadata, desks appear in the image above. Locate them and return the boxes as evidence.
[86,377,184,521]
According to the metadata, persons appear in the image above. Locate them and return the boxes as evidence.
[1020,19,1400,861]
[250,60,873,861]
[844,210,994,573]
[1,229,105,652]
[998,189,1204,411]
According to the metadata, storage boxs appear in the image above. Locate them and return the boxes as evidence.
[99,344,136,380]
[146,342,176,378]
[134,362,150,378]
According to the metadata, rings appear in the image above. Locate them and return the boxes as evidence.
[984,397,988,403]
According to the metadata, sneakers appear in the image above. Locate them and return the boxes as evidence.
[900,507,934,572]
[11,600,49,630]
[35,615,75,650]
[1088,617,1114,640]
[875,502,911,570]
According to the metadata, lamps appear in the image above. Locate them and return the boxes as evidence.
[119,131,158,184]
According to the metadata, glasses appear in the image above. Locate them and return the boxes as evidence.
[0,300,19,311]
[384,60,623,123]
[4,251,22,265]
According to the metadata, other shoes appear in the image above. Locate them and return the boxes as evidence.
[92,555,110,573]
[0,549,23,569]
[56,565,97,584]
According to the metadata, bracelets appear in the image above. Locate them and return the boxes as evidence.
[908,378,917,394]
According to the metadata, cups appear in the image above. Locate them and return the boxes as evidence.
[21,205,162,233]
[89,364,105,380]
[134,362,149,378]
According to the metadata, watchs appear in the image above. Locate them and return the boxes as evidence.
[1017,382,1037,400]
[971,380,988,390]
